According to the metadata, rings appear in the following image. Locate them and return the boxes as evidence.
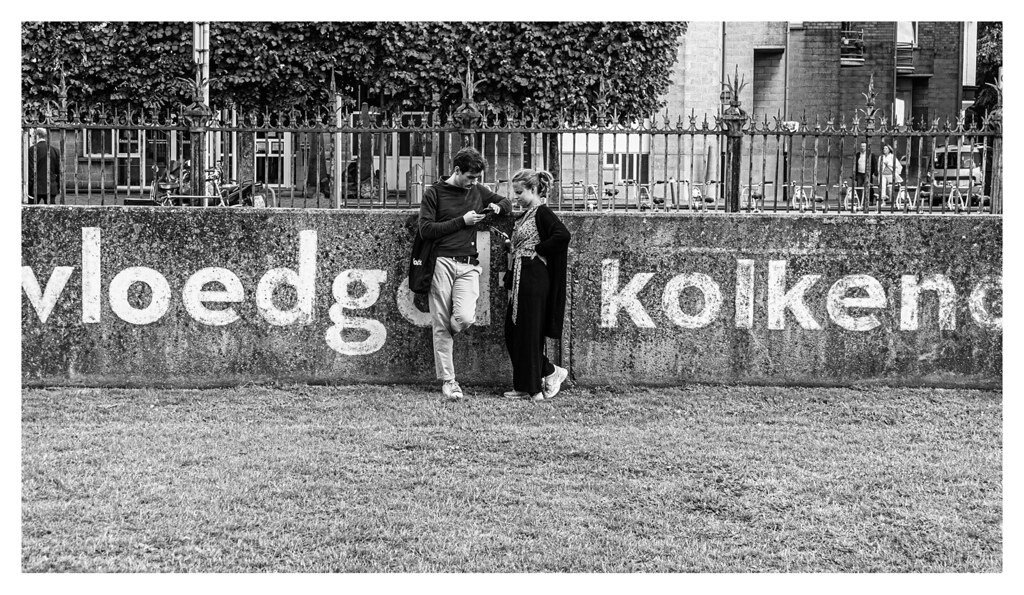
[473,222,475,225]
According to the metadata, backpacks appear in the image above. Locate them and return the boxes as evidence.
[964,104,985,133]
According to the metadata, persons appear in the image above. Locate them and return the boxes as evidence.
[877,143,904,207]
[497,168,571,402]
[28,128,62,205]
[855,141,878,207]
[417,146,513,401]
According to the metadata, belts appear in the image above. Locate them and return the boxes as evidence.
[450,254,480,266]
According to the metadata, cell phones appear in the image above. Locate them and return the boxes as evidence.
[478,208,493,214]
[490,226,508,240]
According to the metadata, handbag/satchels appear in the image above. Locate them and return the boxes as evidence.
[409,228,438,294]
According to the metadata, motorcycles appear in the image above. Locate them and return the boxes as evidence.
[155,157,262,207]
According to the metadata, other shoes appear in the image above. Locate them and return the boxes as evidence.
[504,389,543,401]
[441,380,464,399]
[545,364,568,399]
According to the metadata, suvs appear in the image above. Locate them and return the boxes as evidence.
[920,143,993,207]
